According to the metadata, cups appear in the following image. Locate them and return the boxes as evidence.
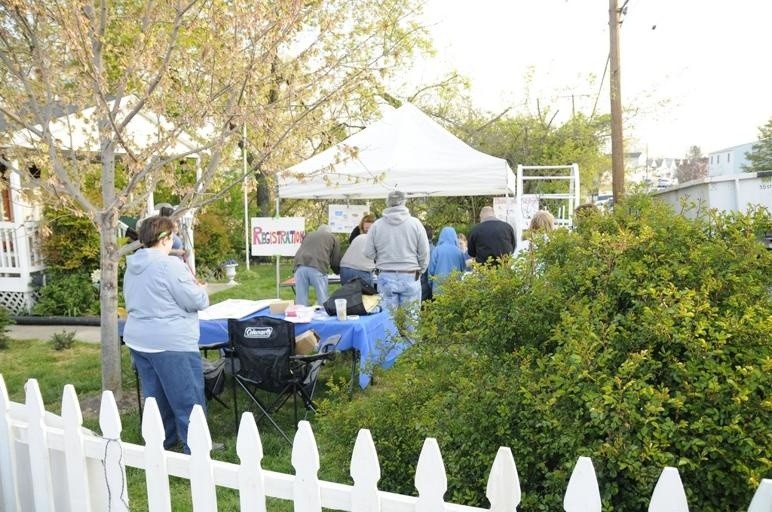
[335,298,347,320]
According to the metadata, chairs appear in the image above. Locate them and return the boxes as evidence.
[136,357,230,424]
[227,316,335,447]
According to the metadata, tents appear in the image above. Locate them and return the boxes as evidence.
[276,100,516,298]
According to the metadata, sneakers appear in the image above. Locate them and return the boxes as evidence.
[213,443,225,451]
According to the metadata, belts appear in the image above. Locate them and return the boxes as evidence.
[380,270,420,281]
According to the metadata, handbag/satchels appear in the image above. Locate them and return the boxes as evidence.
[323,277,382,316]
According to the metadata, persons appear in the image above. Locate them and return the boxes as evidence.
[121,215,226,456]
[350,215,377,243]
[159,207,186,261]
[362,192,430,333]
[292,224,341,307]
[420,224,474,310]
[340,234,375,288]
[575,203,598,218]
[468,205,517,269]
[509,210,556,288]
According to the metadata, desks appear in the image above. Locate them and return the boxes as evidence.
[280,274,378,295]
[118,299,416,421]
